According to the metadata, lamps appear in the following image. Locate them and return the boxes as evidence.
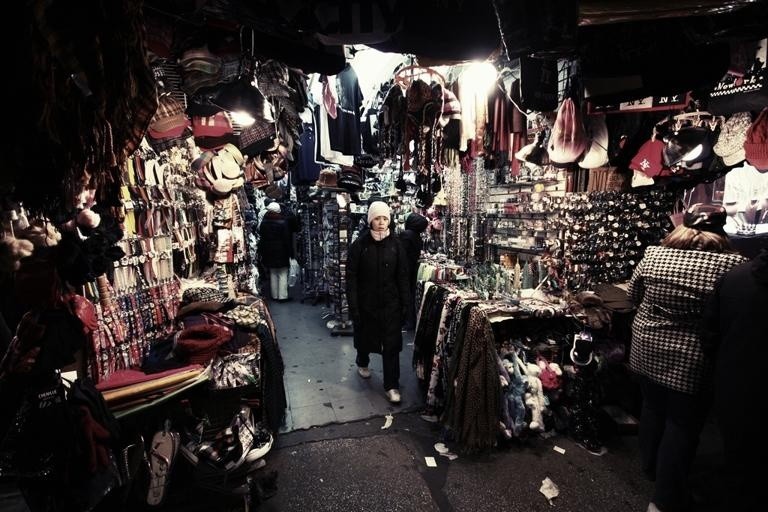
[462,58,501,101]
[513,115,551,167]
[210,25,266,117]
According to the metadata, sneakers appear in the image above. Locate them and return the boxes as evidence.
[384,385,402,404]
[356,364,371,380]
[179,435,204,466]
[246,428,275,463]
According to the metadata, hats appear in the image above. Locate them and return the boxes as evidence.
[267,202,282,214]
[186,87,222,117]
[338,165,363,188]
[147,96,184,131]
[381,80,441,131]
[367,201,391,223]
[747,111,768,174]
[213,83,266,119]
[178,287,225,317]
[146,123,186,139]
[175,43,221,84]
[314,167,337,187]
[188,116,231,137]
[258,59,294,98]
[683,202,728,230]
[631,140,665,179]
[665,115,711,176]
[715,113,753,169]
[243,123,277,156]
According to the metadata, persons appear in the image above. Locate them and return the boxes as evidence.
[627,210,751,512]
[701,247,768,512]
[346,200,410,403]
[256,197,301,303]
[400,213,428,332]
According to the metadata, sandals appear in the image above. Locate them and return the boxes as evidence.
[146,430,181,506]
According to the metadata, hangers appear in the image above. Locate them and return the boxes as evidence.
[393,57,447,92]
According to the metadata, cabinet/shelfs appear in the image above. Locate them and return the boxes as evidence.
[322,201,469,336]
[467,158,565,267]
[296,203,331,307]
